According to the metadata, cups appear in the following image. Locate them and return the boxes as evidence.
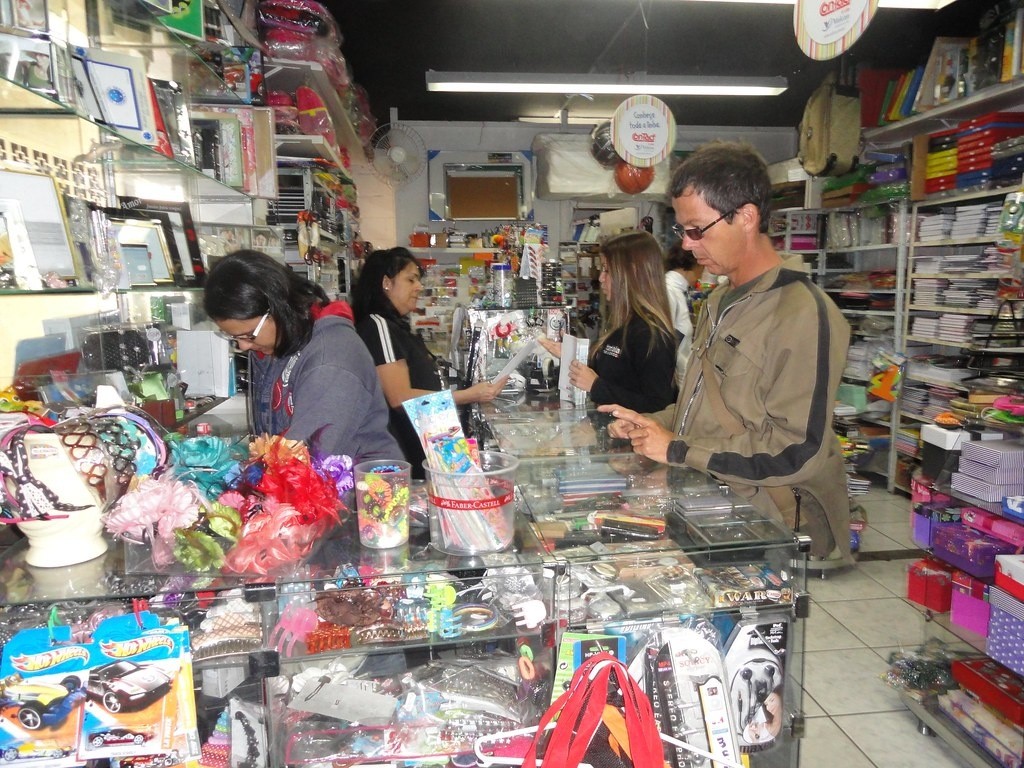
[354,460,412,550]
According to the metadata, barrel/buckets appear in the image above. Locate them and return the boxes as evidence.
[423,450,519,555]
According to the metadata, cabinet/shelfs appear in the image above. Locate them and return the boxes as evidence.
[891,183,1024,498]
[885,331,1024,768]
[0,0,283,435]
[264,57,361,305]
[761,156,909,494]
[560,241,597,305]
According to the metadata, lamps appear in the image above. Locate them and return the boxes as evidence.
[424,70,789,96]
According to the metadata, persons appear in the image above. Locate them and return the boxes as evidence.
[350,247,508,481]
[664,240,704,390]
[597,143,853,558]
[538,232,677,413]
[204,250,406,460]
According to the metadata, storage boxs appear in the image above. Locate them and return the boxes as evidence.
[951,656,1024,728]
[907,500,1024,676]
[920,422,1024,519]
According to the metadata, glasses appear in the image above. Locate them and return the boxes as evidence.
[218,309,271,342]
[672,202,750,241]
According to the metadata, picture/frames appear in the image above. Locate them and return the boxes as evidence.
[101,195,206,287]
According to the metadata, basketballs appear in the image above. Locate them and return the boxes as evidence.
[590,120,656,195]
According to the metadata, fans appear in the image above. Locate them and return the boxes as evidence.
[364,122,428,187]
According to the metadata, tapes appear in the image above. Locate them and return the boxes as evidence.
[542,357,554,377]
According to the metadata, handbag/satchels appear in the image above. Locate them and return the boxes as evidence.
[790,435,857,564]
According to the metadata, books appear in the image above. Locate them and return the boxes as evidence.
[832,204,1004,500]
